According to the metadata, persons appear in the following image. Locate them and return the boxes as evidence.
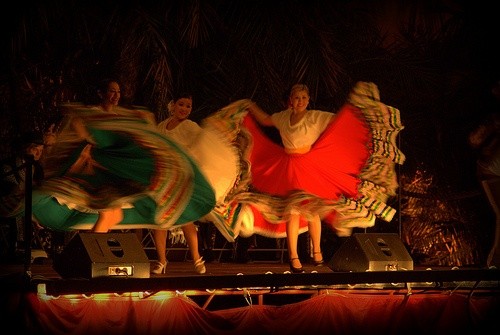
[244,83,338,272]
[150,92,206,274]
[469,77,499,268]
[58,78,153,249]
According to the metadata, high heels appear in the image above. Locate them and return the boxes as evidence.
[313,252,324,267]
[194,256,206,274]
[152,261,167,274]
[288,258,305,274]
